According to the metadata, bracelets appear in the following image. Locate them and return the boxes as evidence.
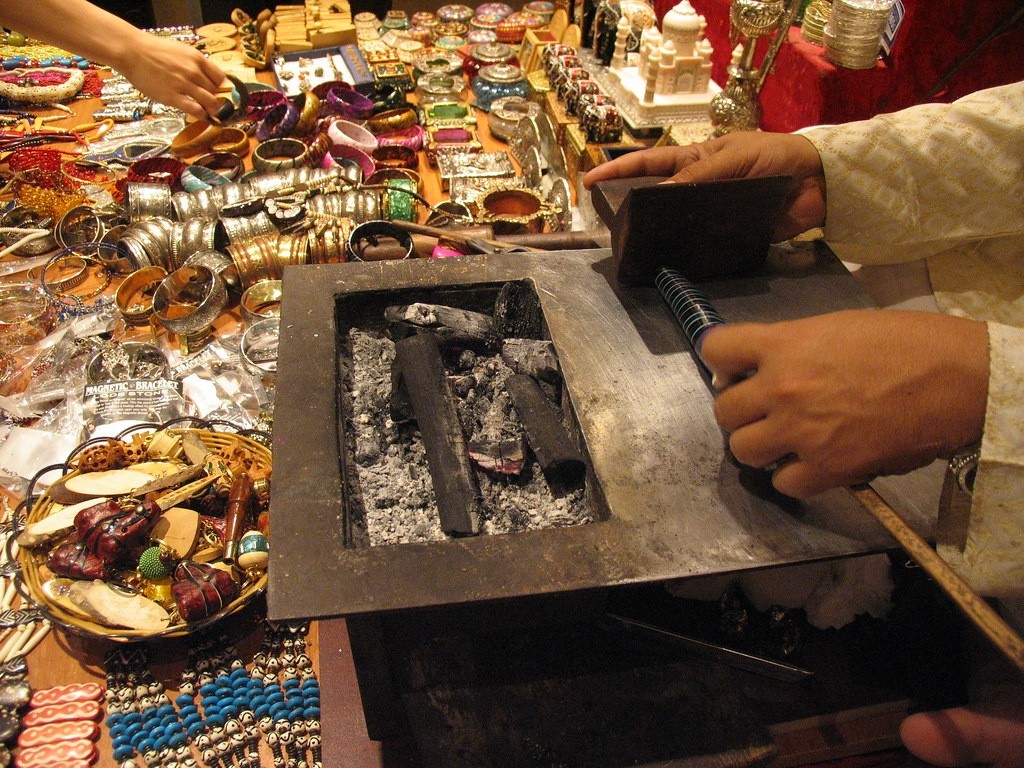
[0,73,471,455]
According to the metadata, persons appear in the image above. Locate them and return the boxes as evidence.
[584,79,1024,768]
[0,0,224,119]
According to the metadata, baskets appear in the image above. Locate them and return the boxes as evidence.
[5,416,272,643]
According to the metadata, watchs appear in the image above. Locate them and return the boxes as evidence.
[950,444,981,496]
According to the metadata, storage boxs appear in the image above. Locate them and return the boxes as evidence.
[269,43,376,103]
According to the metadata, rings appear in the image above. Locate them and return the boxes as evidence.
[275,53,343,92]
